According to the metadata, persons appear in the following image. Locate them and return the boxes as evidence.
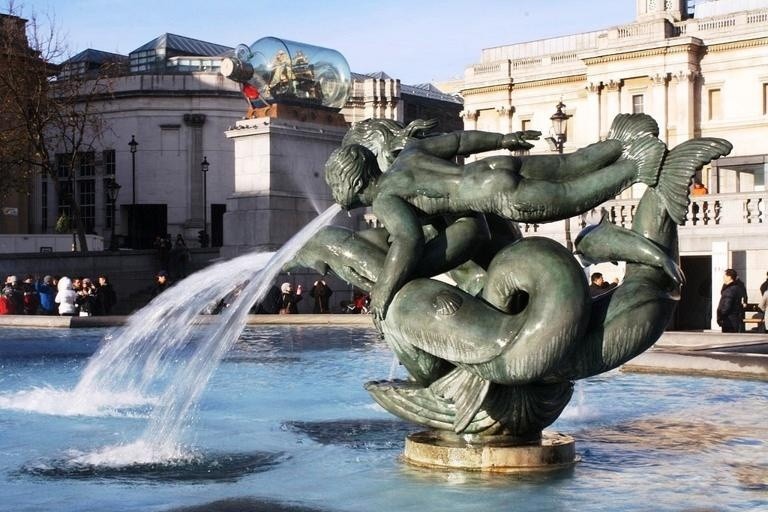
[0,274,117,317]
[716,269,768,333]
[211,279,372,314]
[147,233,192,302]
[690,180,708,225]
[326,113,668,322]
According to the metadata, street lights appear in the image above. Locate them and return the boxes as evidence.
[106,134,140,248]
[198,156,210,248]
[544,102,573,255]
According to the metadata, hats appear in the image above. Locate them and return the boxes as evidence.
[3,272,91,288]
[159,270,168,276]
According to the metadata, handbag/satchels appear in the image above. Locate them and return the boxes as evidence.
[279,308,291,314]
